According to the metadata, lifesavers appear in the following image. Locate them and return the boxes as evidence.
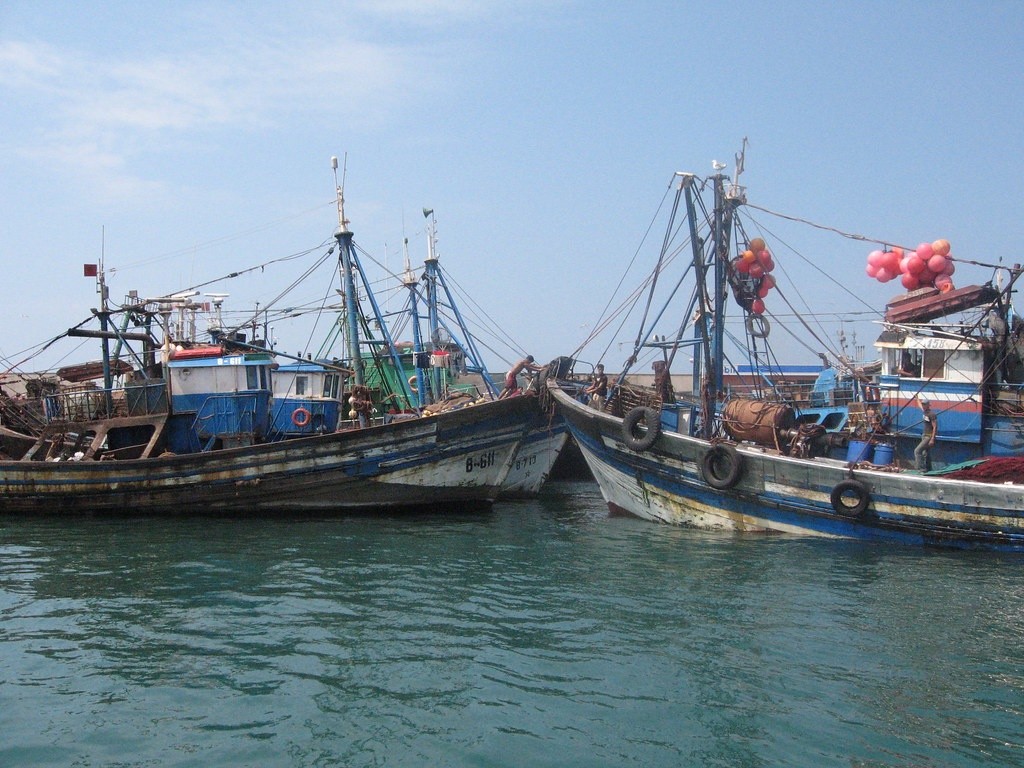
[701,444,744,491]
[621,405,662,453]
[746,313,770,338]
[408,375,419,393]
[830,479,871,518]
[291,408,311,427]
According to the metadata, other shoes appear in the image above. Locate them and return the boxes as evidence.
[918,466,932,472]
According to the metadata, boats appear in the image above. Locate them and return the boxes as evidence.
[542,135,1024,557]
[886,286,940,308]
[0,152,588,515]
[56,358,133,383]
[885,284,999,323]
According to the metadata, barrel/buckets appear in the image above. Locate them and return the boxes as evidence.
[847,440,871,462]
[873,443,894,465]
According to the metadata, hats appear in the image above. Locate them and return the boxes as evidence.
[921,399,928,404]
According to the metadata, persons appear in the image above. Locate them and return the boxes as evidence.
[497,354,547,400]
[584,364,608,412]
[913,394,937,472]
[895,352,917,376]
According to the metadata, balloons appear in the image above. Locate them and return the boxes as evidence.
[866,240,955,293]
[735,239,776,313]
